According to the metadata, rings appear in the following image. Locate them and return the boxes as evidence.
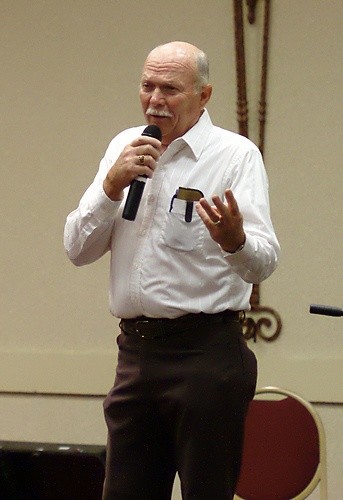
[137,155,144,165]
[213,220,220,225]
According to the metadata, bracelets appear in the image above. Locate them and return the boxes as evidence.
[222,233,247,254]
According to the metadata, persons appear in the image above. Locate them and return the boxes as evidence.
[61,40,281,500]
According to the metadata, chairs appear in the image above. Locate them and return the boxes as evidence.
[233,386,327,500]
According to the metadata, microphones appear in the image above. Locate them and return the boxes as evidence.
[122,125,162,221]
